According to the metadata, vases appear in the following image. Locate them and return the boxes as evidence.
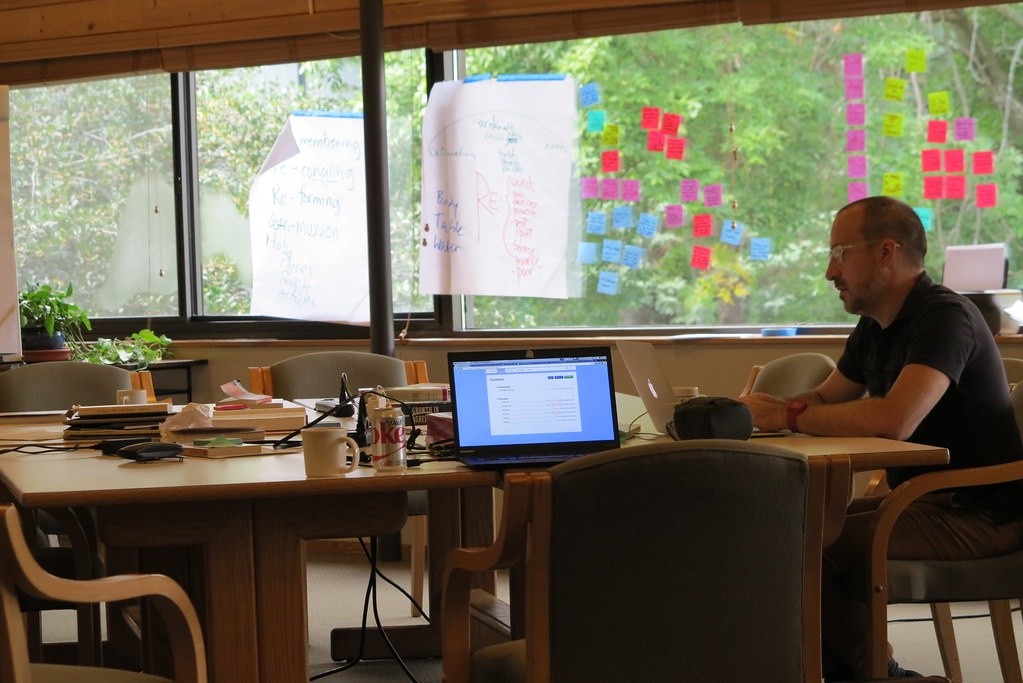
[22,347,71,363]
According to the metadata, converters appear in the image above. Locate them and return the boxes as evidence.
[102,438,152,456]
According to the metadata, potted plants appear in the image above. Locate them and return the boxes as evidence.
[18,282,92,350]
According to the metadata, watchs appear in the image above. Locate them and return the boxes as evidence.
[785,399,807,432]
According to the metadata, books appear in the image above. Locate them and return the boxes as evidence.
[63,378,310,440]
[358,383,453,430]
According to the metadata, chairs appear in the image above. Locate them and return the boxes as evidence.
[0,468,208,683]
[441,439,852,683]
[861,383,1023,683]
[739,352,837,398]
[0,480,103,668]
[247,351,429,643]
[0,360,156,547]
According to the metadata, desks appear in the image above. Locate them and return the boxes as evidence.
[0,400,496,683]
[292,392,948,683]
[113,359,208,404]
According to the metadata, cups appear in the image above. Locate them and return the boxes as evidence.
[117,390,147,404]
[672,386,708,403]
[300,427,360,480]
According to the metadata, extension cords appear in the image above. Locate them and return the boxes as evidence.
[346,427,372,448]
[316,397,355,418]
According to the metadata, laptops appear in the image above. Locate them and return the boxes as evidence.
[447,346,620,469]
[616,340,789,437]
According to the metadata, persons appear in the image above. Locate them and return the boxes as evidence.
[734,195,1023,683]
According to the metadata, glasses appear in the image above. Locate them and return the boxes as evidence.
[828,239,902,265]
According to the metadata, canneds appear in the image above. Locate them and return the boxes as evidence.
[371,407,406,471]
